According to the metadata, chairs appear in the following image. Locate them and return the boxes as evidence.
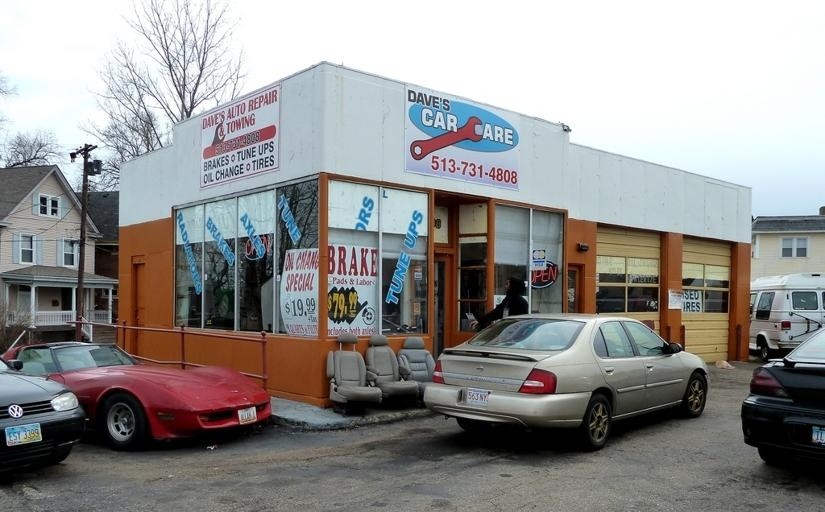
[326,332,383,416]
[396,335,439,395]
[364,332,419,400]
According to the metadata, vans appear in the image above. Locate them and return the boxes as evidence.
[747,272,825,361]
[569,286,657,313]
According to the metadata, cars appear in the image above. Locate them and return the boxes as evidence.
[740,327,825,472]
[427,312,711,450]
[0,356,83,485]
[175,252,237,332]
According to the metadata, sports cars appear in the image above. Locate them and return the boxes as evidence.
[1,338,272,448]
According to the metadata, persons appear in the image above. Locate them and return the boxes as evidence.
[470,279,526,328]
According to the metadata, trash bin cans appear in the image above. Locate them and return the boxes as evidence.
[35,325,77,344]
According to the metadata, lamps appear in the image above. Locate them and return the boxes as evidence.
[578,241,590,251]
[434,215,443,230]
